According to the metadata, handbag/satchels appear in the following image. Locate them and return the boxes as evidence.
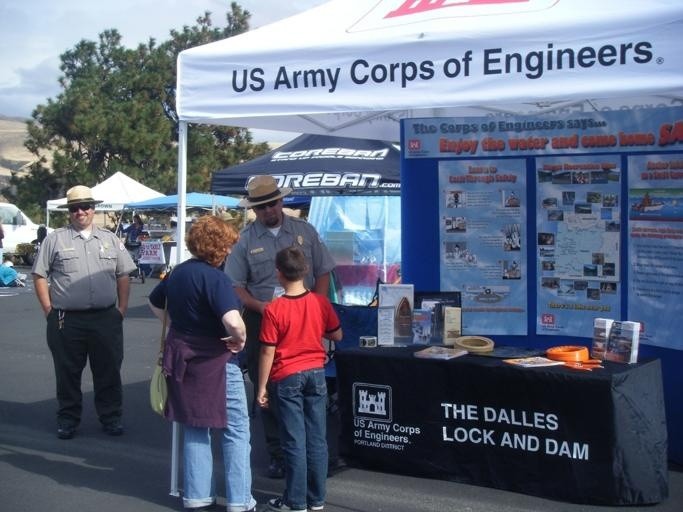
[149,270,173,418]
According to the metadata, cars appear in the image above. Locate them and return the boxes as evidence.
[0,203,54,256]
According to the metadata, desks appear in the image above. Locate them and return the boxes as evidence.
[334,344,667,505]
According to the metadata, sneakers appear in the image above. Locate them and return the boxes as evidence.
[103,424,123,435]
[269,498,307,511]
[194,499,225,509]
[57,427,77,438]
[247,505,269,512]
[307,505,324,511]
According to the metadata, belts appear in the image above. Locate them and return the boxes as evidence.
[52,304,119,314]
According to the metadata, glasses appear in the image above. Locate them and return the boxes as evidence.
[70,204,93,213]
[255,202,281,211]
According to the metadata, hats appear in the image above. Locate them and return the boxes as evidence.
[60,186,101,207]
[239,175,292,207]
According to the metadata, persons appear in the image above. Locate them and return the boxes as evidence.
[1,217,5,266]
[30,185,138,438]
[38,219,48,249]
[119,215,145,280]
[147,214,269,512]
[223,175,335,477]
[255,245,343,512]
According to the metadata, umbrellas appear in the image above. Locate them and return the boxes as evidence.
[168,0,683,498]
[208,134,401,285]
[122,192,245,211]
[45,170,167,228]
[235,195,312,210]
